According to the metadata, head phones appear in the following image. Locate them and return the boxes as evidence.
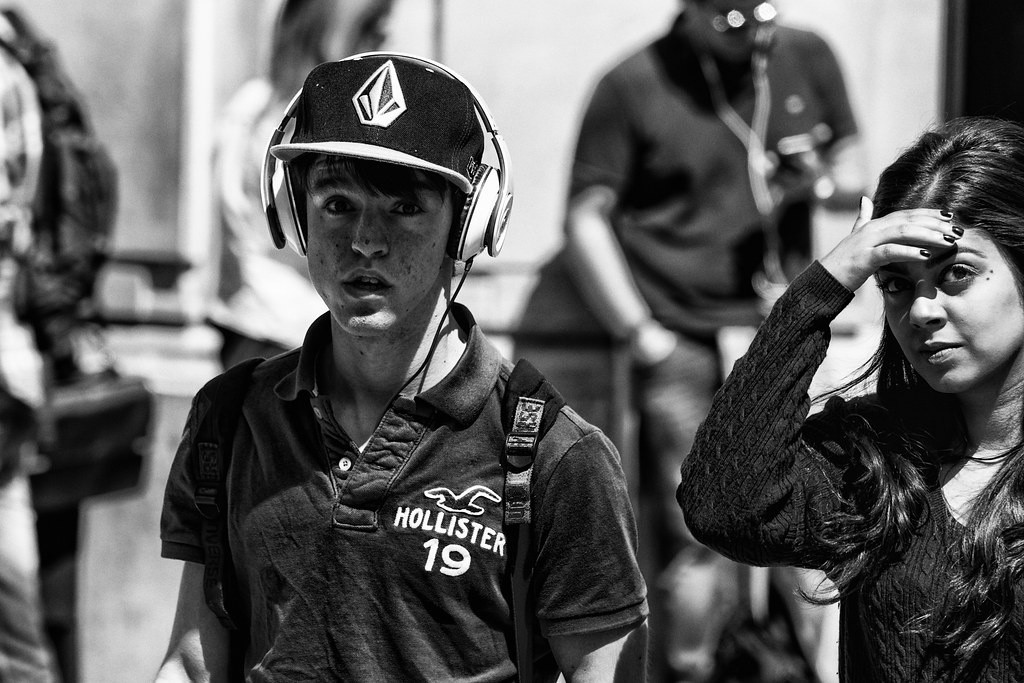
[260,51,513,262]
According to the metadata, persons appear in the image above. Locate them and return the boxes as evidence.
[676,118,1024,683]
[0,11,84,683]
[150,52,650,683]
[566,0,878,683]
[207,0,394,376]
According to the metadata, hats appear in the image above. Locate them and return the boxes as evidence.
[268,54,486,196]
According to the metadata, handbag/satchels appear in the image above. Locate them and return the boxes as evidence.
[37,367,155,511]
